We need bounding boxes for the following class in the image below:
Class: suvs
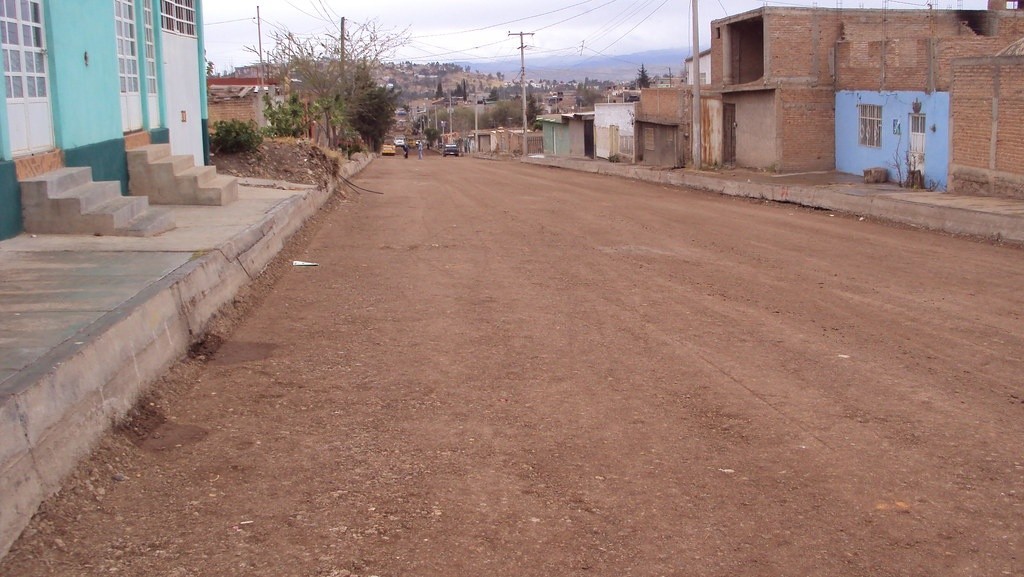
[442,144,460,157]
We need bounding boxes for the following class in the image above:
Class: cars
[397,107,408,115]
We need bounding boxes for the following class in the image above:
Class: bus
[395,134,406,147]
[381,135,396,156]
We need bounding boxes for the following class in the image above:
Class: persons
[417,140,423,158]
[403,142,409,158]
